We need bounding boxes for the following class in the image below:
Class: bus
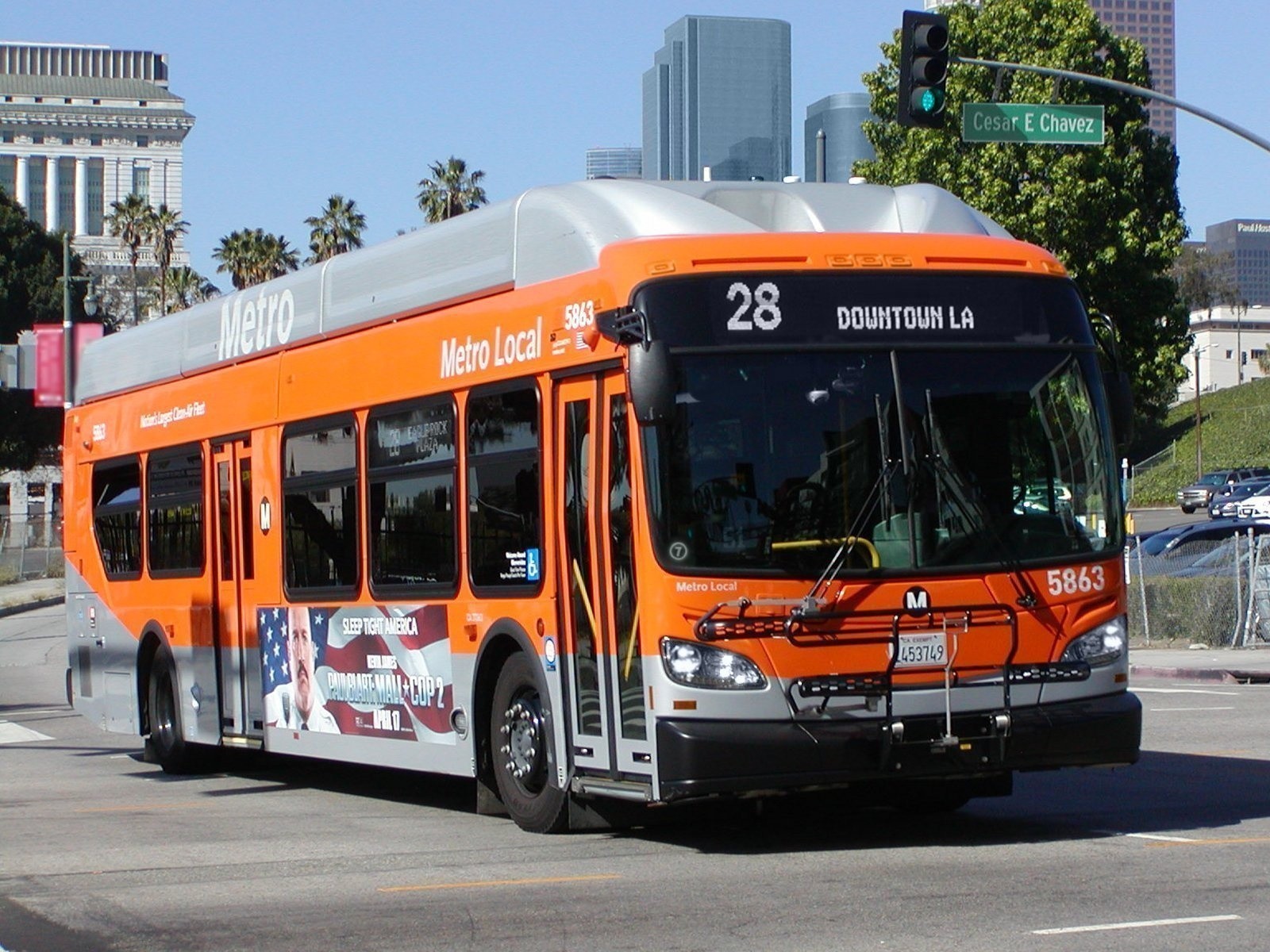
[64,177,1141,837]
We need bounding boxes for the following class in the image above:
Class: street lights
[1195,343,1219,481]
[1237,303,1262,386]
[54,231,99,417]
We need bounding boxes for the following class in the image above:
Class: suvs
[1128,515,1270,577]
[1177,465,1270,514]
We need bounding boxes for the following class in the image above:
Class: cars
[1236,485,1270,519]
[1208,480,1270,521]
[1212,475,1270,500]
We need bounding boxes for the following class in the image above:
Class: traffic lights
[899,7,951,130]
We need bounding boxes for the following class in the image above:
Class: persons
[889,439,979,516]
[262,607,342,734]
